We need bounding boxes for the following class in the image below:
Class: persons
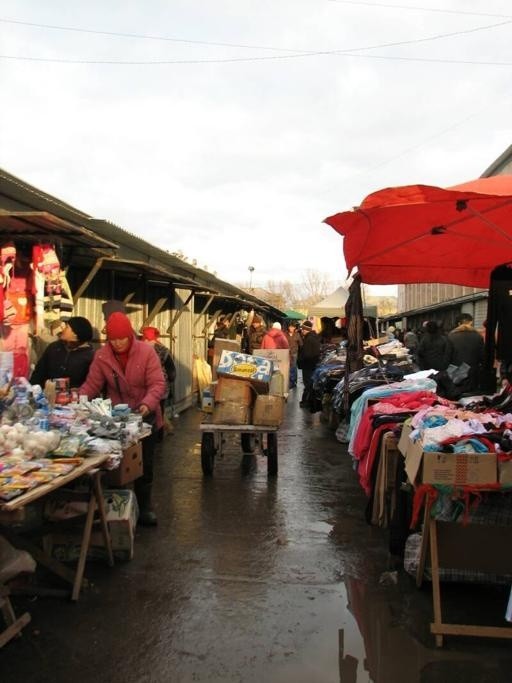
[385,319,455,372]
[439,311,490,395]
[74,310,167,526]
[136,325,178,455]
[206,313,336,409]
[476,319,499,394]
[27,316,95,386]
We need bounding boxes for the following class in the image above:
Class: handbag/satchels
[403,495,512,585]
[142,410,156,454]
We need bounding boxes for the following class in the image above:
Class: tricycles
[198,421,278,476]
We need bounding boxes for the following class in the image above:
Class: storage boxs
[211,348,290,425]
[395,423,511,485]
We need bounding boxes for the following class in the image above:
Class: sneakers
[138,502,158,526]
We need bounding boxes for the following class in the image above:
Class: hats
[253,316,261,322]
[142,327,155,342]
[0,241,16,287]
[272,321,282,330]
[301,321,312,331]
[106,312,132,339]
[68,317,92,341]
[0,299,17,327]
[456,313,472,323]
[32,244,61,273]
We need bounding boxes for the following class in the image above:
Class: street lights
[247,265,255,293]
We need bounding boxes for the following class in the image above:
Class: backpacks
[161,352,170,400]
